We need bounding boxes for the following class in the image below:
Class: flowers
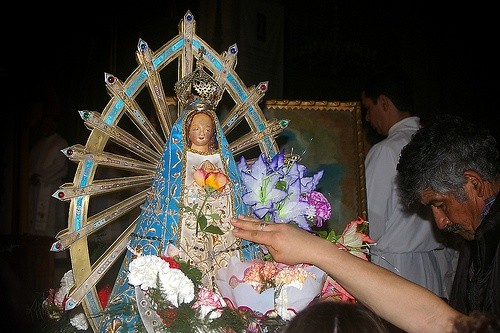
[39,146,399,333]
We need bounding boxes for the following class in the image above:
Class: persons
[99,55,306,333]
[16,111,72,289]
[232,80,500,332]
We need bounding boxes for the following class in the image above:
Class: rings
[260,222,268,231]
